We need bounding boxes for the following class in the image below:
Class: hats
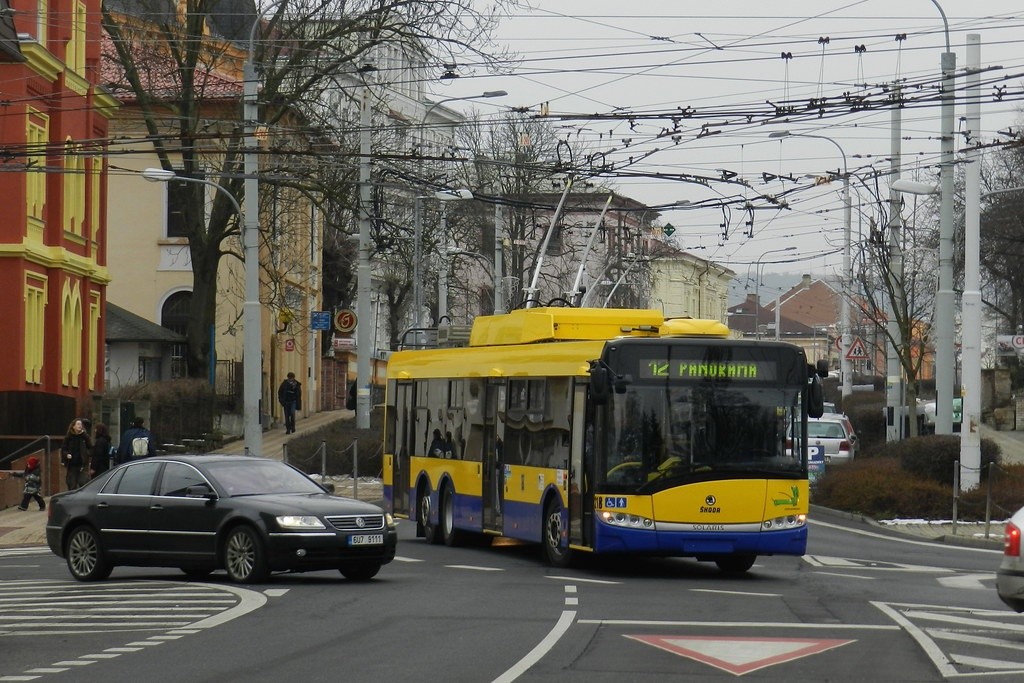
[27,455,40,469]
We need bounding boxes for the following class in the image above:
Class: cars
[820,368,966,436]
[46,457,397,585]
[788,413,860,468]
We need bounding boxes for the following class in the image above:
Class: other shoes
[18,507,27,511]
[286,429,290,434]
[40,505,46,510]
[292,428,296,433]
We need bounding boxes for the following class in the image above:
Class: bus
[382,176,817,576]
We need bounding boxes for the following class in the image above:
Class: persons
[91,423,111,478]
[496,435,504,468]
[119,417,156,464]
[444,432,456,458]
[278,372,302,434]
[427,429,446,458]
[586,399,641,462]
[9,456,46,511]
[63,418,92,490]
[350,378,357,415]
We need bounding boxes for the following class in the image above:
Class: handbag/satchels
[347,398,356,410]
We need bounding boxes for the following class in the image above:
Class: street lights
[635,200,691,310]
[268,309,297,429]
[405,89,508,352]
[769,288,810,343]
[143,165,262,461]
[756,246,798,339]
[768,130,853,408]
[892,177,953,441]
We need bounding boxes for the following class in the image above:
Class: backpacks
[105,439,118,460]
[132,429,149,455]
